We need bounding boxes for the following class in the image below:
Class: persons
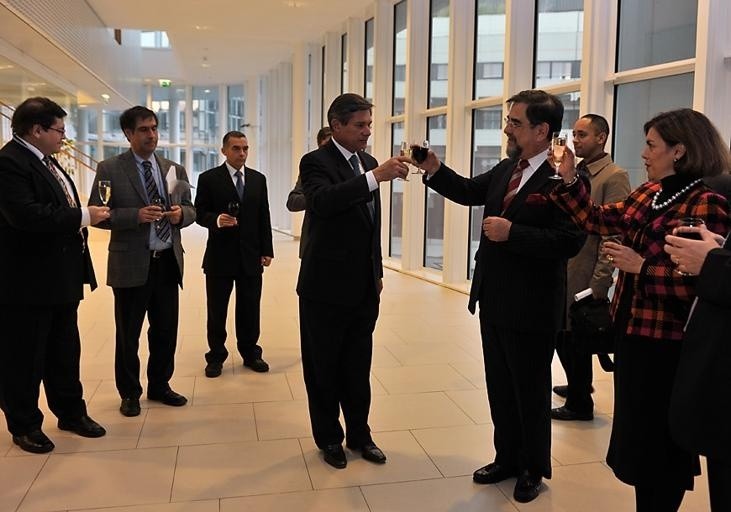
[551,114,632,421]
[296,93,415,468]
[286,127,334,260]
[410,90,590,503]
[664,224,731,512]
[194,131,274,377]
[87,106,196,416]
[0,97,110,454]
[547,108,731,512]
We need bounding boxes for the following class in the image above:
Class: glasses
[49,127,65,136]
[504,118,534,129]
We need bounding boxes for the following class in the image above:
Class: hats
[143,161,170,242]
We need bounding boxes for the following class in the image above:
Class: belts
[153,251,162,258]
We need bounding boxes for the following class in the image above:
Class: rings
[608,256,614,261]
[675,257,680,264]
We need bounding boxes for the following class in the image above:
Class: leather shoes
[58,414,105,437]
[550,386,593,421]
[119,395,140,416]
[355,441,386,462]
[514,467,542,503]
[243,357,269,372]
[13,430,54,453]
[147,390,187,406]
[473,461,518,484]
[323,444,346,468]
[205,362,222,377]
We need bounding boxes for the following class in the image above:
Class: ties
[350,155,374,222]
[44,157,86,253]
[500,160,530,216]
[234,171,244,202]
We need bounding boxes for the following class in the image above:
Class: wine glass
[674,218,706,278]
[396,142,414,182]
[548,132,568,180]
[152,199,165,230]
[410,141,429,175]
[99,180,112,208]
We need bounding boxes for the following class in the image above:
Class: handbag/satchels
[569,294,618,353]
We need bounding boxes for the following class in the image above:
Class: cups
[228,201,240,217]
